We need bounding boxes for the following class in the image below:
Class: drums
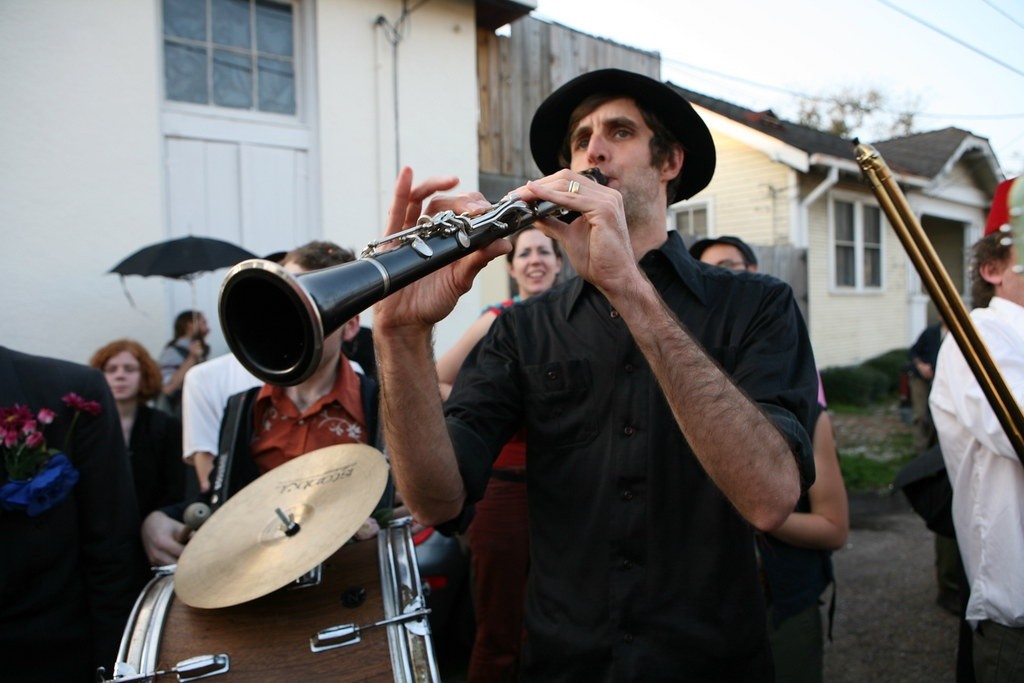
[107,520,445,683]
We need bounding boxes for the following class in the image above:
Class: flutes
[216,166,605,390]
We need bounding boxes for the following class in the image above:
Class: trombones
[849,142,1024,476]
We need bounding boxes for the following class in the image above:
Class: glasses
[717,260,751,269]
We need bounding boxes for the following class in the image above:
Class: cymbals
[169,440,393,612]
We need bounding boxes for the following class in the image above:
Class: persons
[170,249,430,548]
[381,65,828,683]
[152,308,214,417]
[912,172,1023,683]
[1,347,147,683]
[433,218,849,683]
[85,339,186,513]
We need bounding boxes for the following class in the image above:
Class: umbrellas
[104,234,263,316]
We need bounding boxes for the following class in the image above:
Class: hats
[530,69,715,207]
[690,236,757,265]
[984,175,1024,238]
